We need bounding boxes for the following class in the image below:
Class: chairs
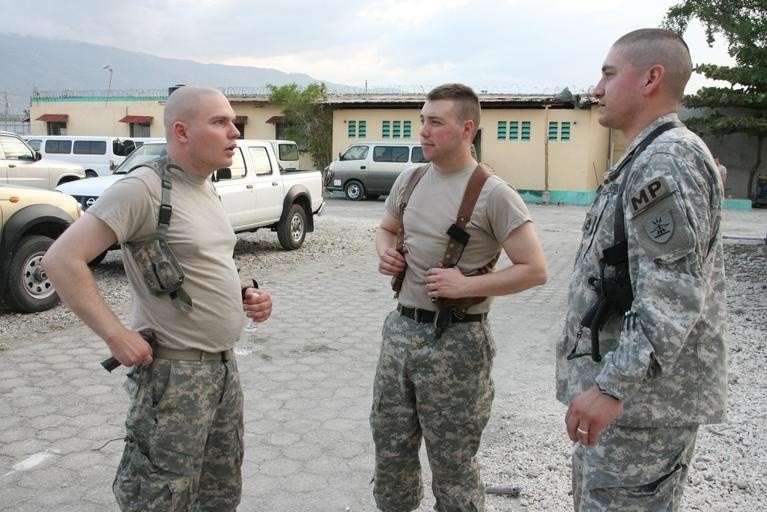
[374,150,391,161]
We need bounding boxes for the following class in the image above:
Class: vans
[322,140,477,202]
[26,137,136,178]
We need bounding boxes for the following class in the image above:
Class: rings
[576,427,589,434]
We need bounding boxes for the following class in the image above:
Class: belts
[151,348,233,362]
[395,302,488,323]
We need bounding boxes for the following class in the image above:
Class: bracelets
[241,278,259,300]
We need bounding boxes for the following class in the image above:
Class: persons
[555,28,725,512]
[369,84,548,512]
[712,152,728,191]
[38,86,272,512]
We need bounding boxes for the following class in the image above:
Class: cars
[0,129,87,195]
[0,181,108,316]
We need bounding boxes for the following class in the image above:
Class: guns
[580,277,635,331]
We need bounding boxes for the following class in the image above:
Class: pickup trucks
[53,139,324,251]
